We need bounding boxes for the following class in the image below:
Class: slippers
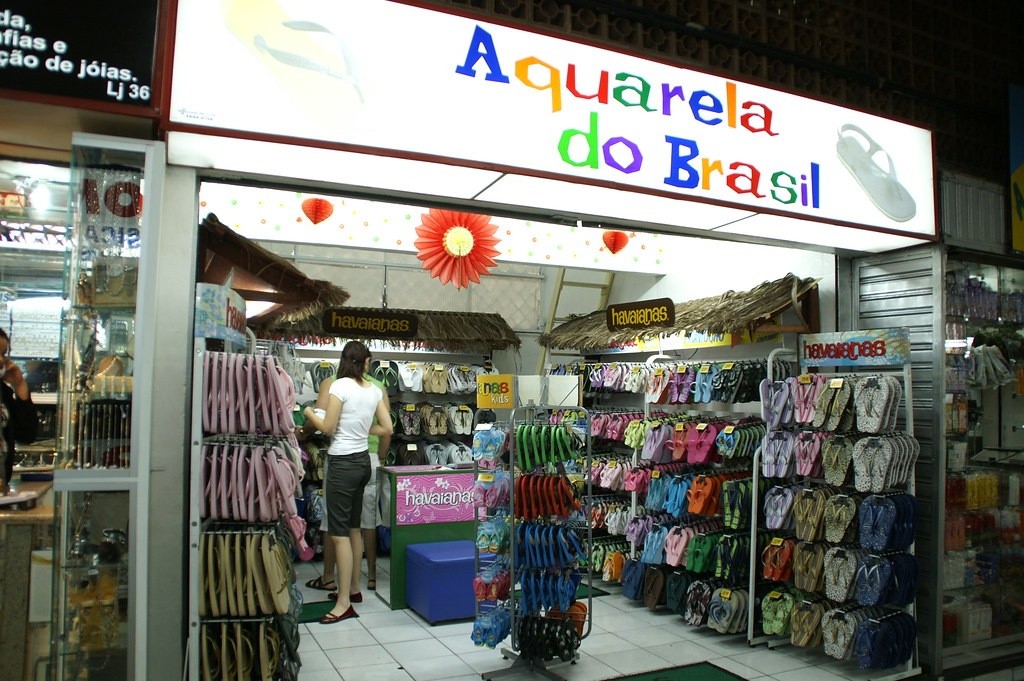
[471,426,587,662]
[551,362,765,633]
[196,352,313,680]
[759,373,921,672]
[281,341,499,480]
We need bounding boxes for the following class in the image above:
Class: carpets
[510,582,611,603]
[603,660,749,681]
[297,599,360,623]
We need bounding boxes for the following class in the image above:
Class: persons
[305,340,393,623]
[0,327,38,499]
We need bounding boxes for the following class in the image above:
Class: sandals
[305,575,337,591]
[367,579,376,590]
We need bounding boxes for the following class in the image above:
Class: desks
[0,482,73,681]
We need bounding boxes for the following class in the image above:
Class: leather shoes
[328,593,362,603]
[320,604,354,624]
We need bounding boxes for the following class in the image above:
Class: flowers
[415,207,504,289]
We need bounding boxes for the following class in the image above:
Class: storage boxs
[29,549,52,621]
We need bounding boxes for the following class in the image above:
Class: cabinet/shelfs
[246,305,520,560]
[850,247,1024,681]
[49,135,168,681]
[547,276,822,647]
[184,212,351,681]
[744,328,923,681]
[474,373,594,681]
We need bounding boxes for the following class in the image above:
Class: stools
[404,540,496,628]
